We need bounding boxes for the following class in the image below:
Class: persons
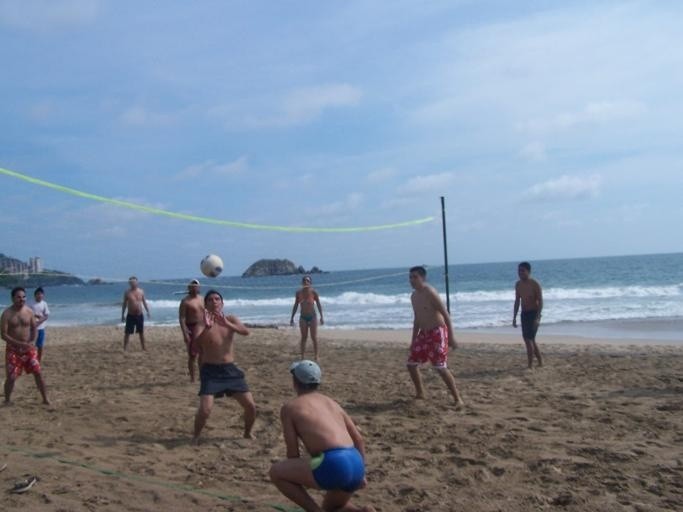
[264,358,369,511]
[176,278,205,382]
[28,288,49,364]
[510,258,545,371]
[0,286,55,407]
[118,276,151,353]
[284,273,326,362]
[189,289,258,443]
[402,263,464,410]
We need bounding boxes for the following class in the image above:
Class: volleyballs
[200,255,223,278]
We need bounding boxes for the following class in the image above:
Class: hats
[187,279,200,288]
[290,359,321,384]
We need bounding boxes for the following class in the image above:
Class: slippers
[11,476,37,493]
